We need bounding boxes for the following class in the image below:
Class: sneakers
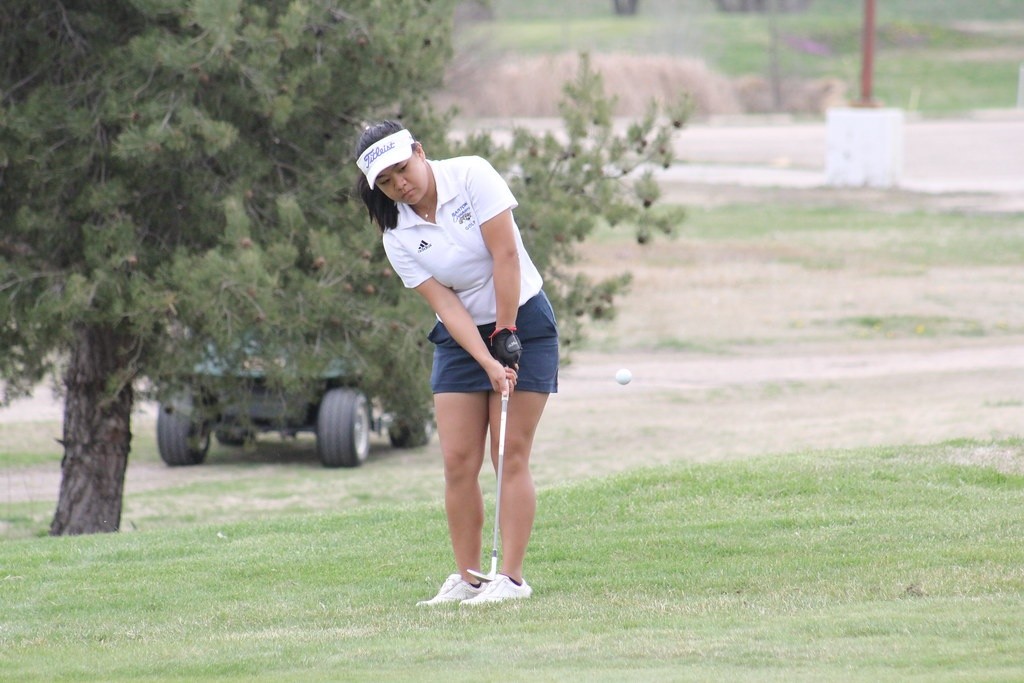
[415,573,489,608]
[459,573,533,609]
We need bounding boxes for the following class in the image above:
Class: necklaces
[401,164,436,220]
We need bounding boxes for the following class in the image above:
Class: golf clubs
[466,363,512,584]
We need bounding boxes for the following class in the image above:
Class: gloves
[489,327,523,374]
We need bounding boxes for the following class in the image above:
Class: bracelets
[495,325,518,331]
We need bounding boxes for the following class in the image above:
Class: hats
[356,128,415,191]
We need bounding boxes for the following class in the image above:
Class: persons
[355,120,560,609]
